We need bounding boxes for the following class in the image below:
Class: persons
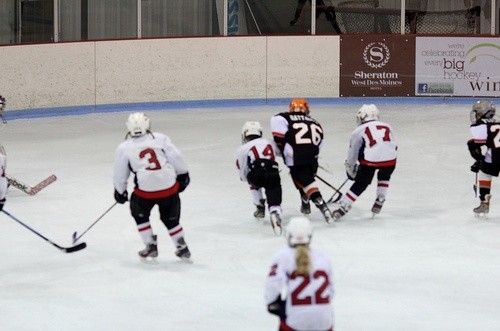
[0,96,9,211]
[266,217,336,331]
[236,121,284,236]
[270,99,335,225]
[467,98,500,213]
[110,112,191,259]
[331,104,398,221]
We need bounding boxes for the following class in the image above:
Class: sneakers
[315,196,335,225]
[253,199,266,224]
[300,203,311,214]
[175,236,192,262]
[473,194,491,220]
[331,205,347,223]
[371,196,385,219]
[138,234,158,261]
[270,209,283,238]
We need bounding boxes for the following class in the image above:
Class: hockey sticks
[315,174,342,203]
[2,209,87,254]
[73,201,117,244]
[328,178,349,202]
[6,173,58,197]
[473,172,478,197]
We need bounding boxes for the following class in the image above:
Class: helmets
[241,121,263,145]
[125,112,150,136]
[472,99,496,119]
[290,98,310,115]
[357,103,380,123]
[286,216,311,247]
[0,95,6,117]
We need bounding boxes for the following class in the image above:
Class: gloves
[346,172,355,181]
[268,293,286,319]
[176,172,190,193]
[115,188,129,204]
